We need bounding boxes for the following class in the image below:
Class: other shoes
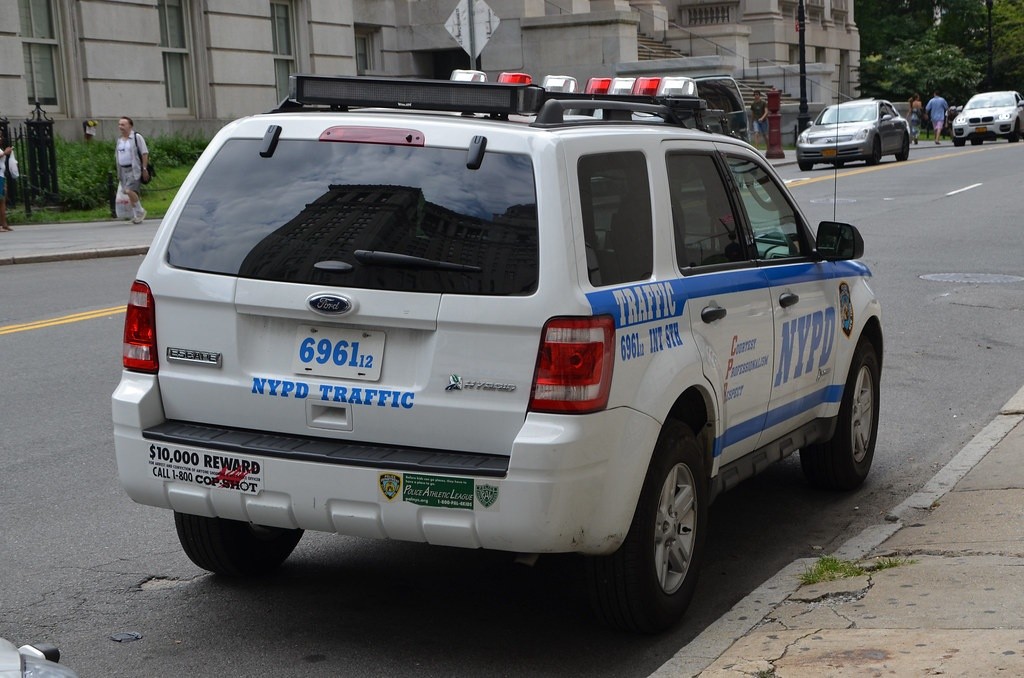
[0,226,13,232]
[135,208,147,222]
[128,218,142,224]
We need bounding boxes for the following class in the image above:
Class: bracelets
[143,168,147,171]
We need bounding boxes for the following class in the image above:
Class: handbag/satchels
[139,164,156,185]
[9,151,19,178]
[115,180,135,218]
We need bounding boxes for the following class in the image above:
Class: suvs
[109,59,887,638]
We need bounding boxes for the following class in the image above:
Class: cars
[795,96,911,172]
[951,91,1024,146]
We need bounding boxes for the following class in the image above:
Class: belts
[121,164,132,168]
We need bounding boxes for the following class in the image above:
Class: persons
[926,91,959,145]
[909,93,922,145]
[115,117,149,224]
[750,90,769,150]
[0,127,13,232]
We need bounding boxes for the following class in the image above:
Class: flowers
[84,120,98,126]
